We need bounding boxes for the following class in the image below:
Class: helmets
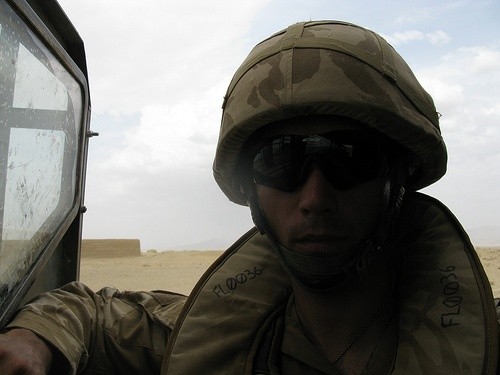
[210,20,446,204]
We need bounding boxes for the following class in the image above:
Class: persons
[0,21,500,375]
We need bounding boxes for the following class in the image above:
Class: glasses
[242,130,389,191]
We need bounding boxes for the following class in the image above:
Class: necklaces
[330,258,397,366]
